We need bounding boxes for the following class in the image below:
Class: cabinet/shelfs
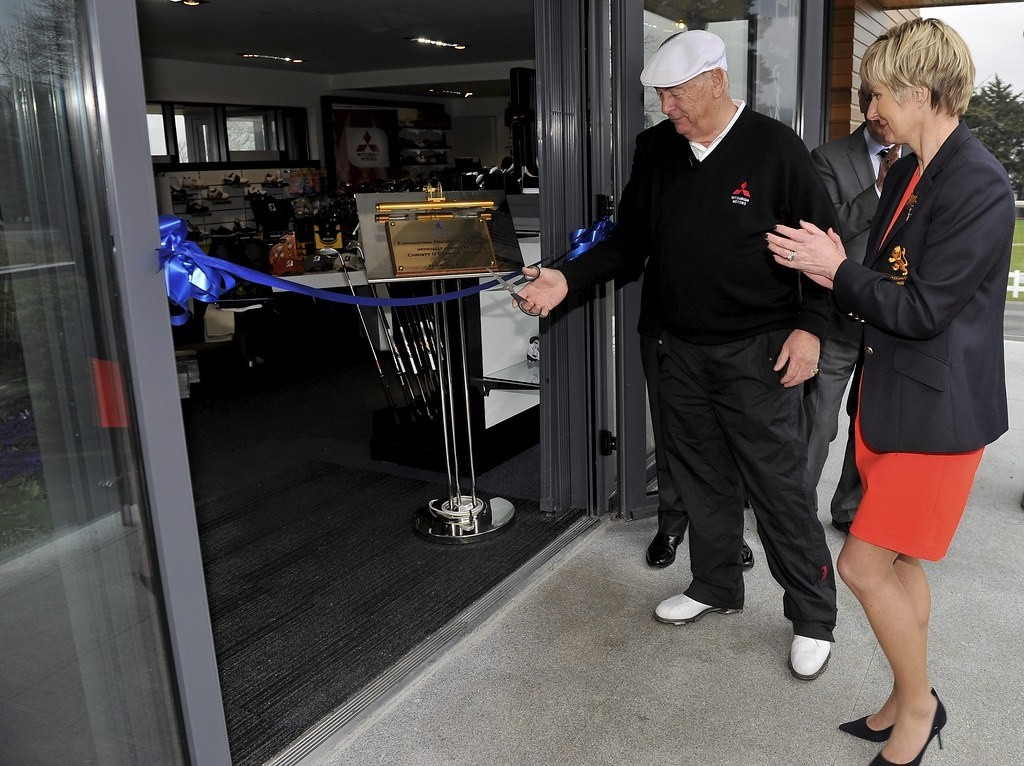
[403,118,453,179]
[152,158,330,260]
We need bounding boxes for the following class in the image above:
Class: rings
[786,250,796,262]
[810,368,819,374]
[887,161,890,169]
[885,156,890,163]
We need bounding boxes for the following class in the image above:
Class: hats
[639,30,727,87]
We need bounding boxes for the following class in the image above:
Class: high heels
[869,687,947,766]
[840,696,896,743]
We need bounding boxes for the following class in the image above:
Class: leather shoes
[654,594,742,625]
[832,519,852,535]
[788,634,831,681]
[741,539,754,570]
[646,530,685,568]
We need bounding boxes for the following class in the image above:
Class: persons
[801,53,924,539]
[512,29,853,682]
[635,242,755,571]
[760,16,1016,766]
